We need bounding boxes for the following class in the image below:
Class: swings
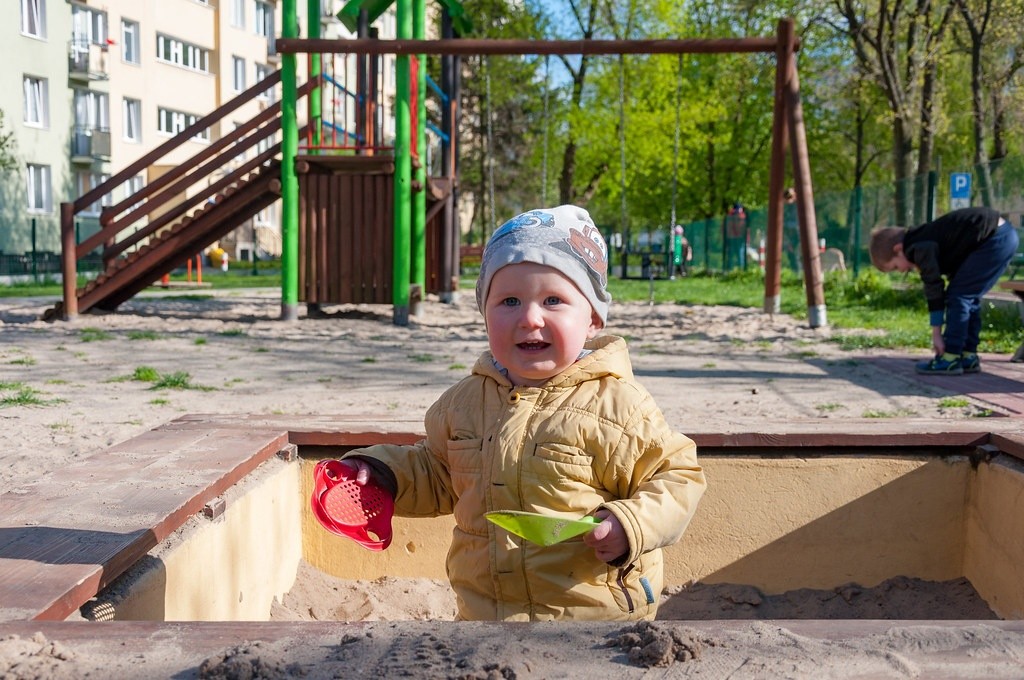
[479,54,552,234]
[619,53,683,281]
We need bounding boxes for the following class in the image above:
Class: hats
[473,203,612,327]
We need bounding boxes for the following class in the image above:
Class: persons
[326,204,708,622]
[869,206,1019,375]
[641,224,693,280]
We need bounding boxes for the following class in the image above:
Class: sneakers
[962,351,981,372]
[915,351,966,375]
